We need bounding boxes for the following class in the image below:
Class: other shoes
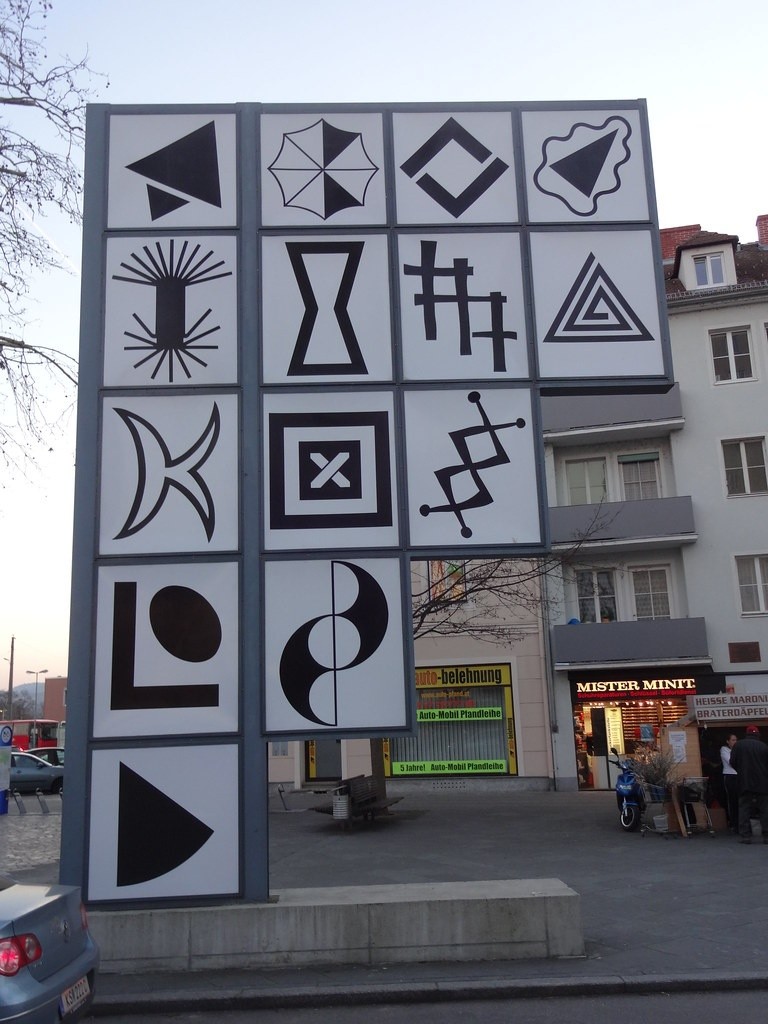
[763,835,768,844]
[735,834,753,844]
[727,827,737,836]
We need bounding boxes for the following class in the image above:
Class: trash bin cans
[333,795,351,819]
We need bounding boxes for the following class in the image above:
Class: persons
[730,724,768,844]
[720,732,751,836]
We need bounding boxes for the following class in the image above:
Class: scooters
[607,747,648,832]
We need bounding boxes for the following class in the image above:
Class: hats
[746,725,761,733]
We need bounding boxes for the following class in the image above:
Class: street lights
[26,670,49,718]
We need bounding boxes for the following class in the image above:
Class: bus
[0,719,59,753]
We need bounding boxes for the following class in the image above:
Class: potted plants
[600,607,609,623]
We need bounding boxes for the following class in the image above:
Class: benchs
[349,774,404,828]
[307,774,364,828]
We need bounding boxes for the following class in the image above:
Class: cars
[10,752,64,795]
[23,746,66,767]
[0,872,103,1024]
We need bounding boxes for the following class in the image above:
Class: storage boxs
[652,814,668,830]
